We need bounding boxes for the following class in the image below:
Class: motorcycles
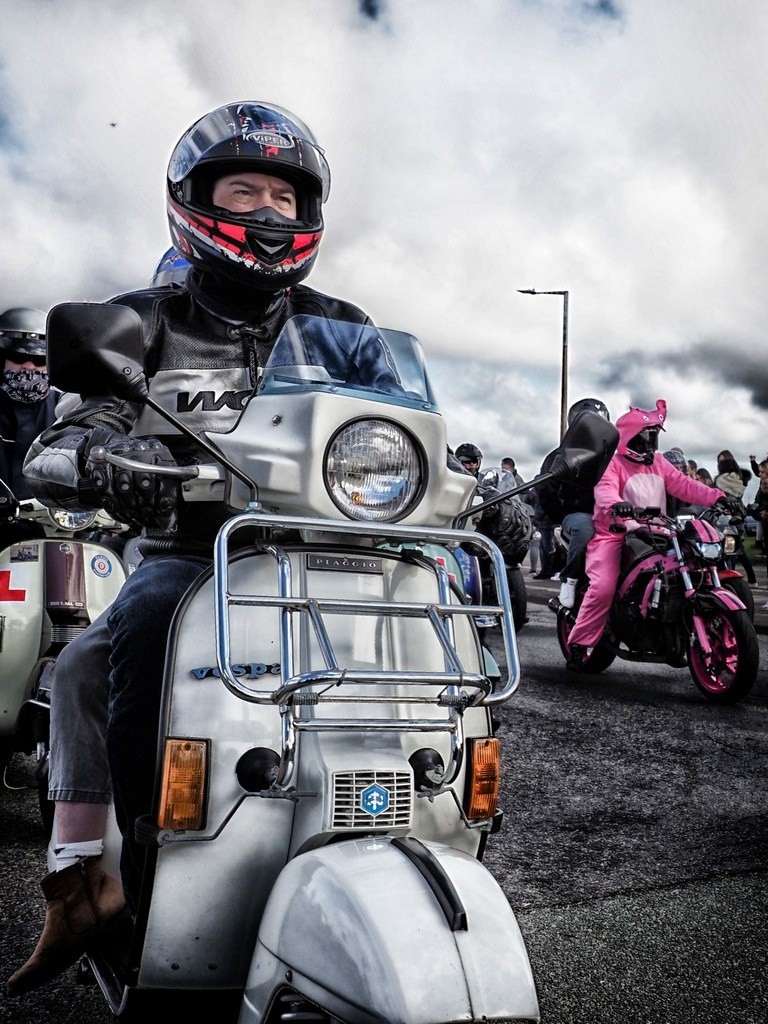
[0,292,767,1024]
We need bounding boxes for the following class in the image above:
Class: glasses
[0,349,49,368]
[463,458,477,464]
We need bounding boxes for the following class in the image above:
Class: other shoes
[528,569,537,576]
[748,582,758,588]
[533,573,551,580]
[564,645,584,682]
[8,851,125,997]
[550,572,561,581]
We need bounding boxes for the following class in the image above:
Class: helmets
[163,103,331,287]
[628,421,658,455]
[664,450,688,477]
[568,399,610,429]
[456,443,483,475]
[0,307,49,357]
[152,248,192,284]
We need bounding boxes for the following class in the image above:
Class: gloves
[79,424,181,528]
[717,496,740,517]
[458,485,535,569]
[612,502,633,518]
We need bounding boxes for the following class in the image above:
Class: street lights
[516,288,569,450]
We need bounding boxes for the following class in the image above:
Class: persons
[663,447,768,589]
[447,443,566,578]
[0,102,425,999]
[542,398,738,670]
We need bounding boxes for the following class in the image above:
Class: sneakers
[559,577,578,608]
